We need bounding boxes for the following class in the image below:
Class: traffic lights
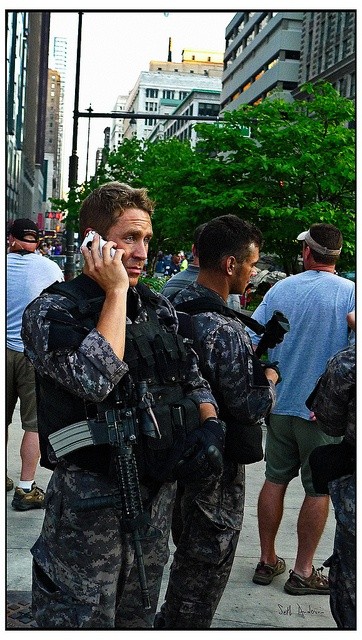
[44,211,62,221]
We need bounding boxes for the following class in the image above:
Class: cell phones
[80,230,122,269]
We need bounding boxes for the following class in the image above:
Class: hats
[296,228,344,257]
[7,217,39,243]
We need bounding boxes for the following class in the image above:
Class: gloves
[263,359,282,384]
[178,416,227,478]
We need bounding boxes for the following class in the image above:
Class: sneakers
[283,565,331,596]
[5,475,14,491]
[252,554,286,586]
[11,482,45,510]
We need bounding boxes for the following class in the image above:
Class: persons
[19,179,229,630]
[6,219,66,512]
[160,222,243,312]
[306,344,356,628]
[164,249,188,276]
[171,213,284,629]
[37,238,62,255]
[244,221,354,594]
